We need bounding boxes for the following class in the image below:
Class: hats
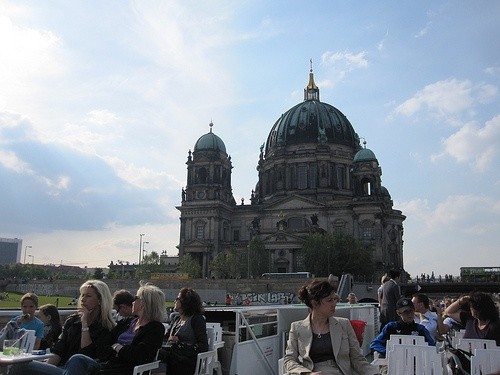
[395,299,414,308]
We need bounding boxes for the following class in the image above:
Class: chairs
[379,334,500,375]
[16,322,225,375]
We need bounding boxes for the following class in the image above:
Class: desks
[0,351,55,364]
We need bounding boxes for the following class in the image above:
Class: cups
[3,340,20,355]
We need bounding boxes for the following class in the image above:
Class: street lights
[24,245,34,264]
[139,233,149,264]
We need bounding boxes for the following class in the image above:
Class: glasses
[176,297,180,301]
[117,306,121,313]
[134,296,140,301]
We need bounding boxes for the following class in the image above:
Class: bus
[460,266,500,282]
[262,271,313,279]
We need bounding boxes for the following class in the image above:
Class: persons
[0,280,210,375]
[226,293,249,305]
[369,268,500,375]
[282,280,382,375]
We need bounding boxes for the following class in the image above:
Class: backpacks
[445,344,482,375]
